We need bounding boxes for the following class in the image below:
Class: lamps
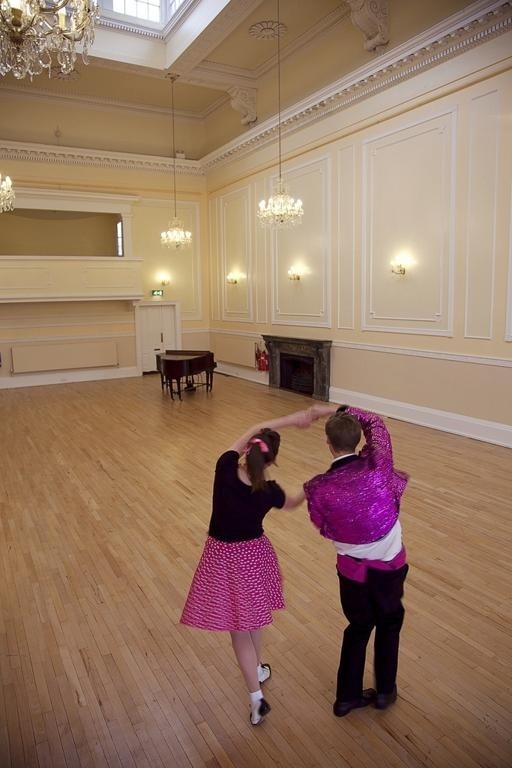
[1,176,16,213]
[257,1,305,230]
[0,1,100,83]
[391,254,407,277]
[157,270,170,286]
[288,261,306,281]
[158,73,195,249]
[228,269,245,285]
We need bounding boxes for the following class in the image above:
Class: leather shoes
[332,684,397,717]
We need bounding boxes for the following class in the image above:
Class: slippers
[259,663,271,685]
[249,699,271,726]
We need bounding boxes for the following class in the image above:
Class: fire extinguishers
[256,349,269,370]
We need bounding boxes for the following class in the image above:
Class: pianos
[156,350,217,401]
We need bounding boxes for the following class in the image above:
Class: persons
[180,410,313,728]
[302,404,411,717]
